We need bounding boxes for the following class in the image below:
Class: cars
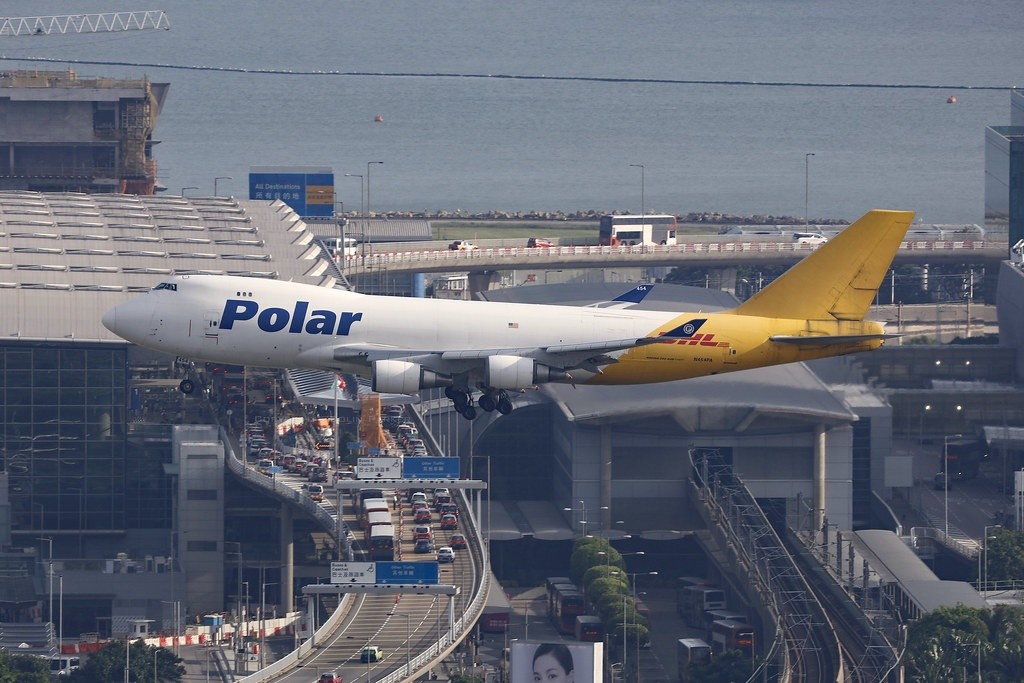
[527,237,556,248]
[194,362,469,683]
[449,240,478,252]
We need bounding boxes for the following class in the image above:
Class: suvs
[792,232,828,248]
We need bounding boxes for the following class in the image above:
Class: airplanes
[99,205,919,423]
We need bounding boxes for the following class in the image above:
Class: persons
[532,644,575,683]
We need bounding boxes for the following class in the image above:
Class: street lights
[318,190,338,263]
[979,536,996,595]
[368,160,383,244]
[629,164,645,254]
[344,174,365,268]
[562,501,659,683]
[945,433,962,545]
[805,153,816,233]
[983,524,1002,601]
[34,536,54,647]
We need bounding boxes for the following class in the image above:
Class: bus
[599,213,677,253]
[480,576,759,683]
[323,237,359,260]
